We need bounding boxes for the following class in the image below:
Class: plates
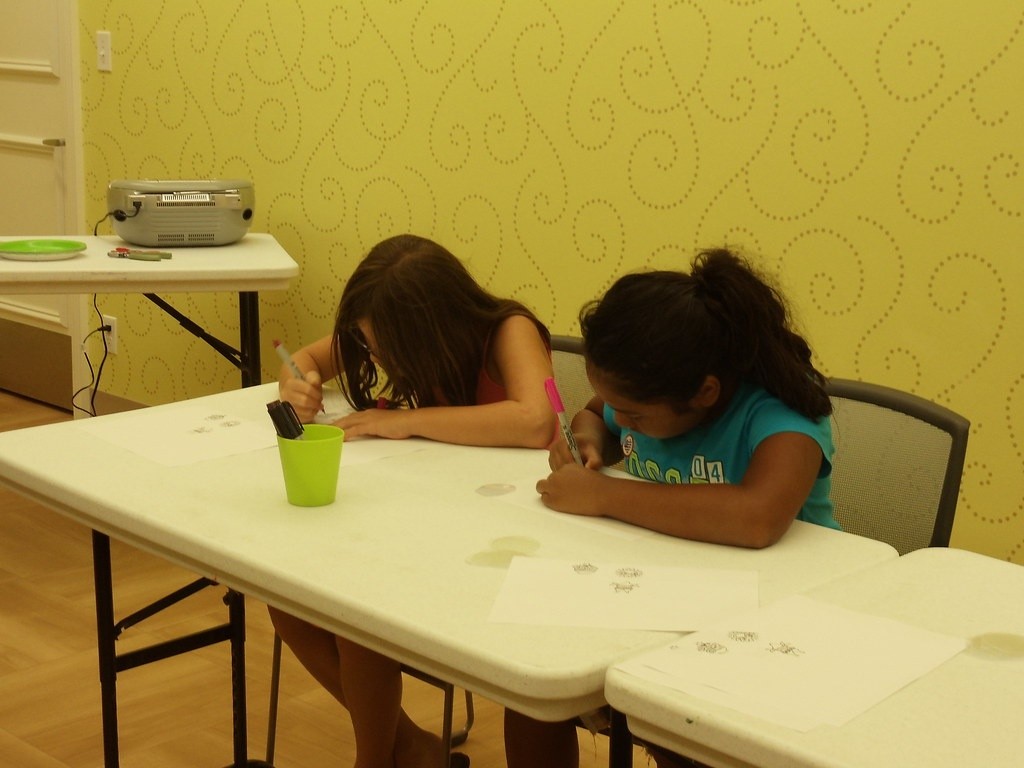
[0,239,87,261]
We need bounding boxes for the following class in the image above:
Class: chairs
[265,336,596,768]
[575,377,970,768]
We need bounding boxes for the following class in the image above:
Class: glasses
[346,327,383,362]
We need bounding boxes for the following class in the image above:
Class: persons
[267,236,556,767]
[503,249,843,768]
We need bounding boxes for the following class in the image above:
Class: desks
[0,234,300,389]
[606,545,1024,768]
[0,380,900,768]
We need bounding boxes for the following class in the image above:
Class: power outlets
[101,314,123,352]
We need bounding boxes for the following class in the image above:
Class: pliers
[108,247,173,260]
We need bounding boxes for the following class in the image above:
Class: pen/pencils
[266,397,306,442]
[272,338,326,416]
[544,376,585,468]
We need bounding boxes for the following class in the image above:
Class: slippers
[450,752,471,768]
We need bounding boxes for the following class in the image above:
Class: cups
[276,424,345,508]
